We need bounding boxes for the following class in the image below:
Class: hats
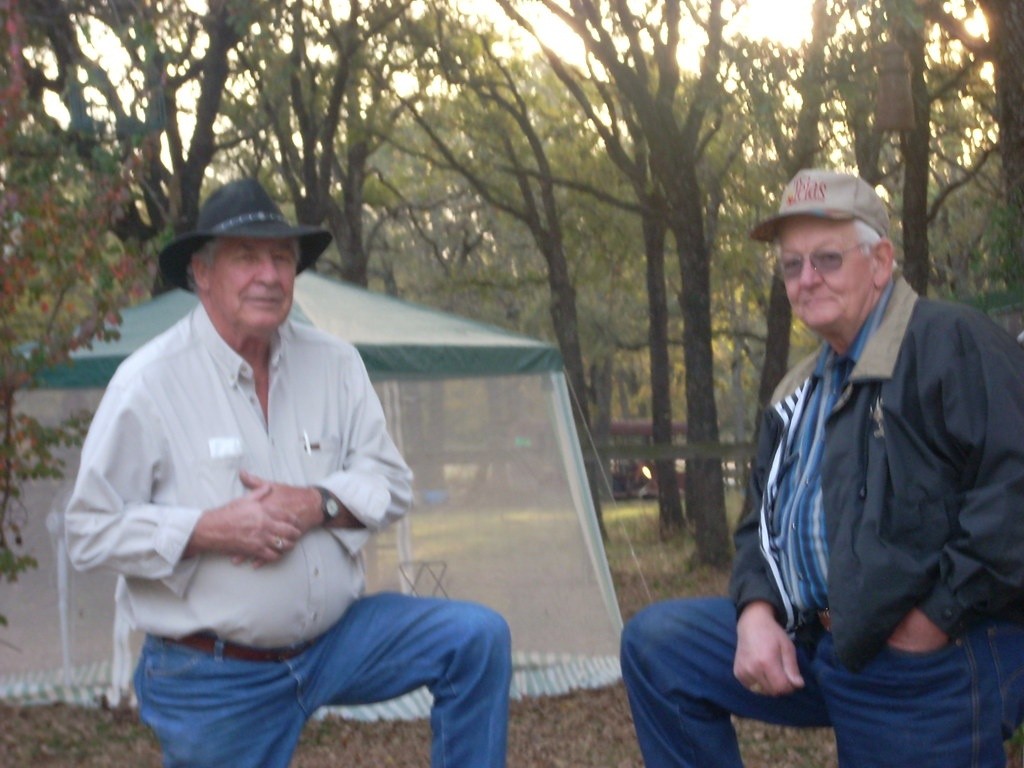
[752,169,890,244]
[159,178,333,294]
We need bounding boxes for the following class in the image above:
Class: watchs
[314,485,338,526]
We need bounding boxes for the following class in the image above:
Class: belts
[803,608,832,634]
[163,632,322,662]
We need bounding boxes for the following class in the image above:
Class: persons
[0,178,512,767]
[619,168,1024,768]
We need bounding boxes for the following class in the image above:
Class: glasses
[775,241,870,279]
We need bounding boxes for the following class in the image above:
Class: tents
[0,265,628,724]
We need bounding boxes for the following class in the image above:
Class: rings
[751,680,765,694]
[275,536,285,554]
